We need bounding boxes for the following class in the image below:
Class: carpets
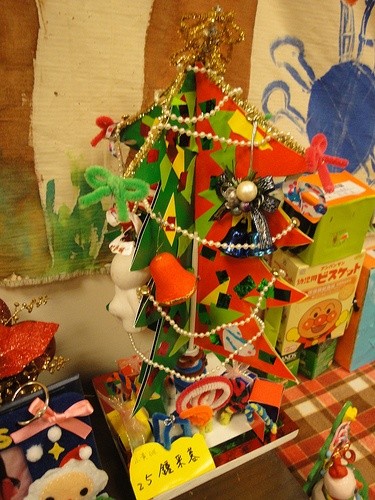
[273,362,375,500]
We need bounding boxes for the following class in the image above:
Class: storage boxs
[260,170,375,392]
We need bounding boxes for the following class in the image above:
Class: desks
[92,353,299,500]
